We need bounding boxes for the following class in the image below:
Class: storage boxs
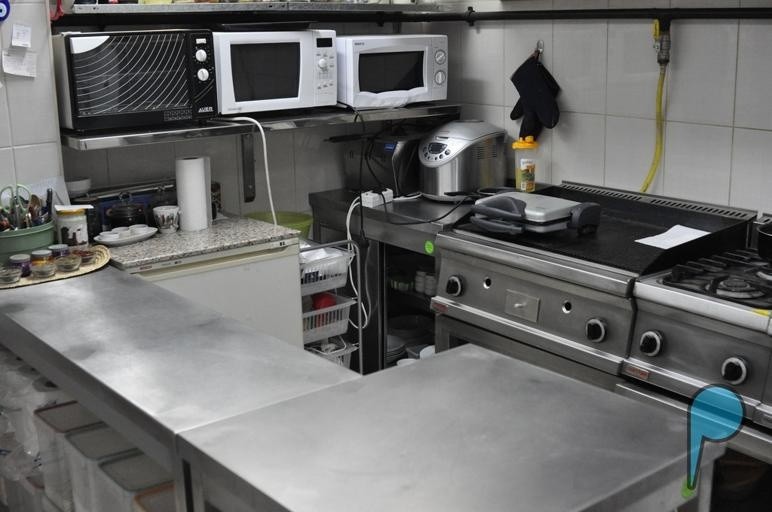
[28,399,177,508]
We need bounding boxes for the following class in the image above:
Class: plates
[93,225,160,246]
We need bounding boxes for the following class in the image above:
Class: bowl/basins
[243,210,314,242]
[129,223,149,235]
[0,265,22,284]
[71,250,96,264]
[27,261,57,279]
[99,231,120,241]
[54,255,82,271]
[383,263,443,369]
[63,176,92,199]
[112,227,130,237]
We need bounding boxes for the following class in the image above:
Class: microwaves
[337,35,450,113]
[50,30,218,134]
[212,31,338,116]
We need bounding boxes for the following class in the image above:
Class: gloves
[507,54,562,141]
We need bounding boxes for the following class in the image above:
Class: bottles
[30,249,52,264]
[47,244,69,258]
[512,135,539,194]
[55,208,88,253]
[8,253,31,277]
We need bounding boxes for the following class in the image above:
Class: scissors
[1,186,31,229]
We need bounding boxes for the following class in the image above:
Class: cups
[152,205,181,234]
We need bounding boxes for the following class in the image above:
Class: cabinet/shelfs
[297,237,372,375]
[54,0,464,155]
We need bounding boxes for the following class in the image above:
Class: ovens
[430,246,633,391]
[616,295,772,511]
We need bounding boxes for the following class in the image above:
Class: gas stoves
[659,242,772,313]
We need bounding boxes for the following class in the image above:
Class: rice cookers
[416,117,511,204]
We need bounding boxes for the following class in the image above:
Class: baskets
[300,290,357,347]
[297,234,357,299]
[303,337,360,370]
